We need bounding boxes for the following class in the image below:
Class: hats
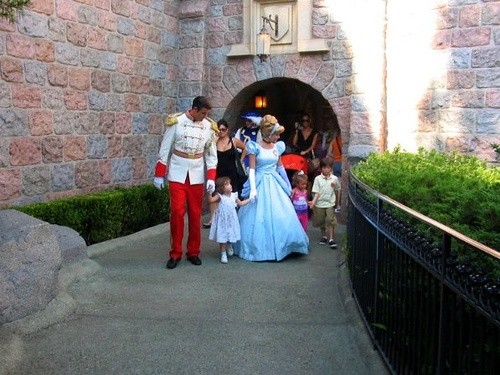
[240,112,262,126]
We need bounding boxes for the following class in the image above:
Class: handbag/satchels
[309,158,320,174]
[235,150,245,179]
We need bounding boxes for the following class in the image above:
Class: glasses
[219,127,227,132]
[301,119,309,123]
[198,110,209,117]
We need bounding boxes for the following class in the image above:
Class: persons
[241,114,293,260]
[289,170,312,233]
[154,96,218,269]
[231,112,342,213]
[310,159,341,247]
[202,118,247,227]
[207,177,251,263]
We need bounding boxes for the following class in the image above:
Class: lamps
[256,15,278,62]
[254,91,267,108]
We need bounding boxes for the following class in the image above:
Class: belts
[173,148,204,159]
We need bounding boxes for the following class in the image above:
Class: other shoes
[327,240,338,247]
[226,243,234,256]
[167,256,181,268]
[319,235,327,244]
[220,250,228,263]
[187,256,201,265]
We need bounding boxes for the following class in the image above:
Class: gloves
[248,167,258,203]
[206,179,215,194]
[278,165,294,197]
[153,176,166,190]
